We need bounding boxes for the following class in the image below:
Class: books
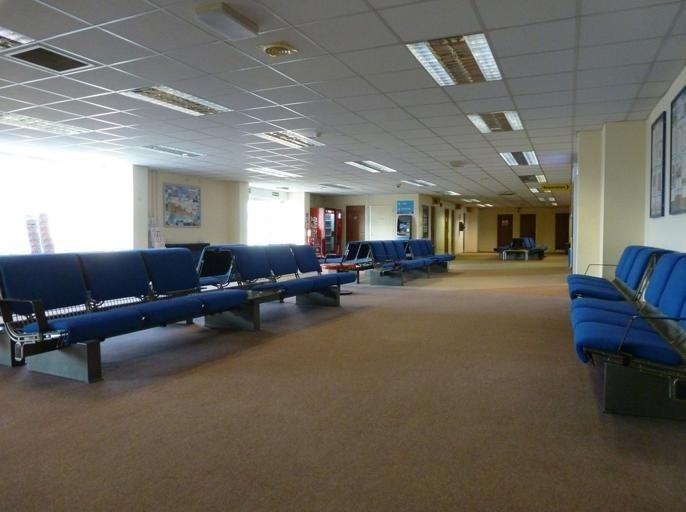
[150,228,165,248]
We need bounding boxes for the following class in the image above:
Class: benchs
[316,240,456,286]
[164,239,358,331]
[1,248,247,381]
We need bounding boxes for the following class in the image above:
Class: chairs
[493,235,548,261]
[566,245,686,421]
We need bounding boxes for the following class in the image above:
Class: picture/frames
[668,85,685,215]
[648,110,666,219]
